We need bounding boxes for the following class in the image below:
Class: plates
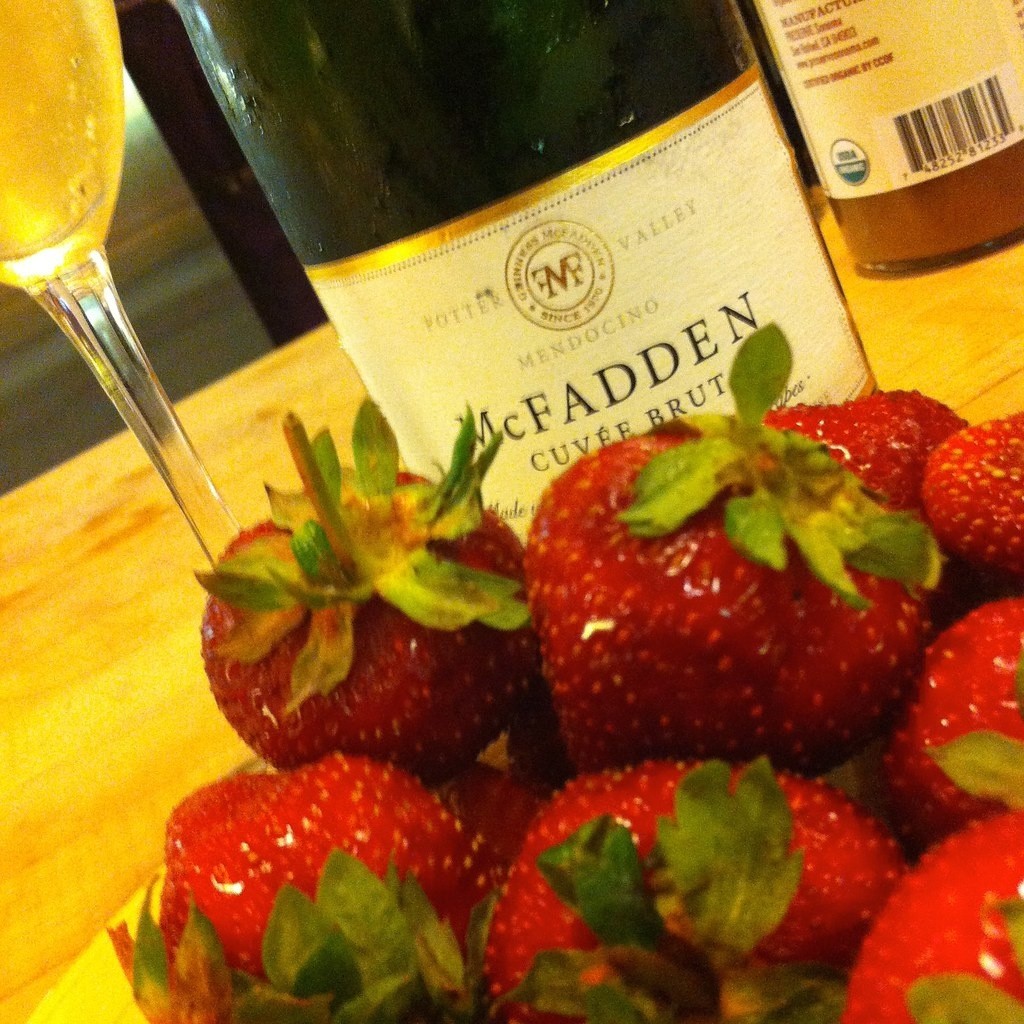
[26,866,171,1024]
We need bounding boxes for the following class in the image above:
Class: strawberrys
[144,390,1024,1024]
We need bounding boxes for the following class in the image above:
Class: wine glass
[0,0,237,570]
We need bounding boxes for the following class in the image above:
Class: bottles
[746,0,1024,282]
[175,0,877,528]
